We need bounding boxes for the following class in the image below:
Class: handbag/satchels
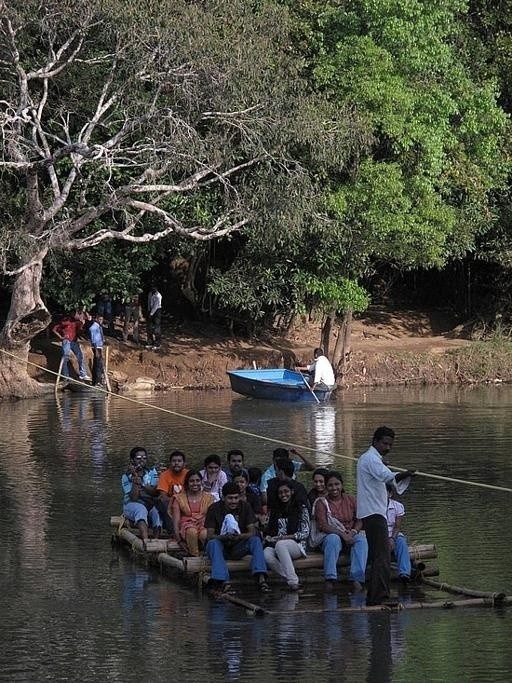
[308,517,346,547]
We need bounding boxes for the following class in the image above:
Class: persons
[52,281,163,388]
[121,445,413,598]
[294,347,336,392]
[355,425,419,605]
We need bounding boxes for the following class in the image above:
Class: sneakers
[290,585,304,593]
[400,575,411,587]
[349,581,363,590]
[325,580,337,590]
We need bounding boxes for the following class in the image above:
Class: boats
[225,368,338,401]
[109,513,511,618]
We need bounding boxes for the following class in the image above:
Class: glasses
[132,455,148,459]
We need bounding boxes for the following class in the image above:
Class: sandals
[222,583,237,595]
[260,582,273,592]
[365,596,400,606]
